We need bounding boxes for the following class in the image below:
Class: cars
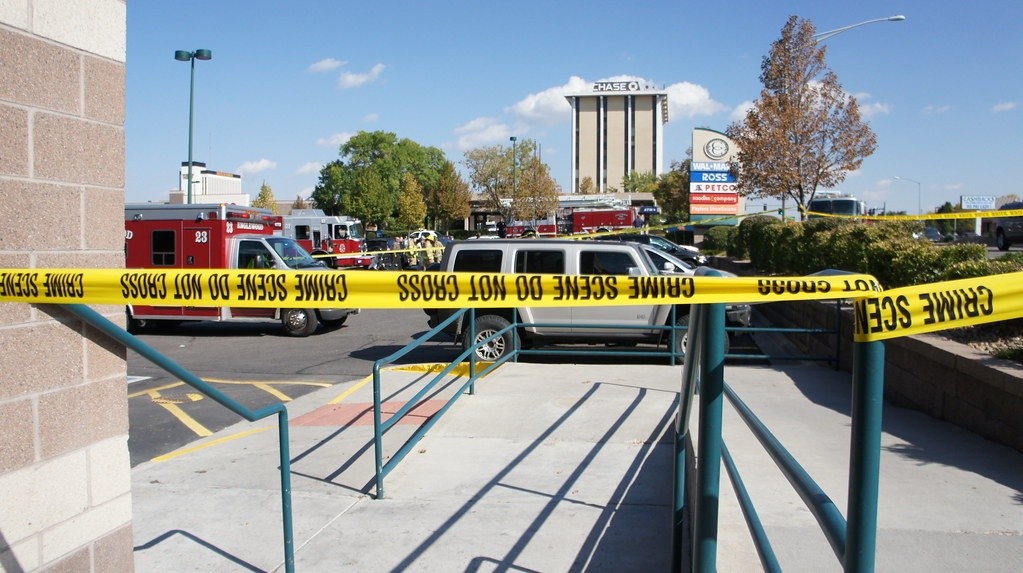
[923,227,944,244]
[368,239,393,263]
[987,202,1023,251]
[399,229,453,247]
[587,233,710,273]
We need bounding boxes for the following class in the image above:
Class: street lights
[174,48,212,204]
[893,176,921,216]
[781,14,906,221]
[509,135,517,198]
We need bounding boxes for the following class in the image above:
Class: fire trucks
[496,206,636,238]
[123,203,373,338]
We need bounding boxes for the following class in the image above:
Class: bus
[808,196,871,220]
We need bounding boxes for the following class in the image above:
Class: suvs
[424,233,755,367]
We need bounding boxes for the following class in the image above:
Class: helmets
[427,235,434,241]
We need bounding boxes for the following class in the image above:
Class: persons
[633,215,644,235]
[386,234,444,273]
